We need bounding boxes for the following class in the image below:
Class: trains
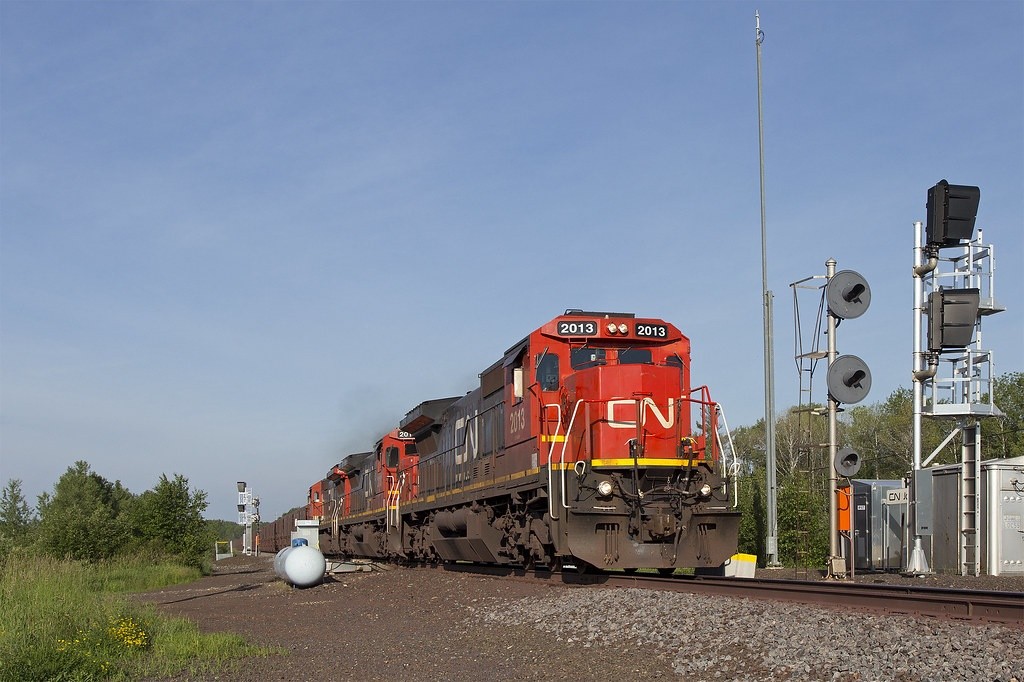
[233,308,744,576]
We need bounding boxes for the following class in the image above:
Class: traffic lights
[923,180,980,247]
[834,447,861,477]
[828,268,873,320]
[827,356,873,402]
[925,285,1009,352]
